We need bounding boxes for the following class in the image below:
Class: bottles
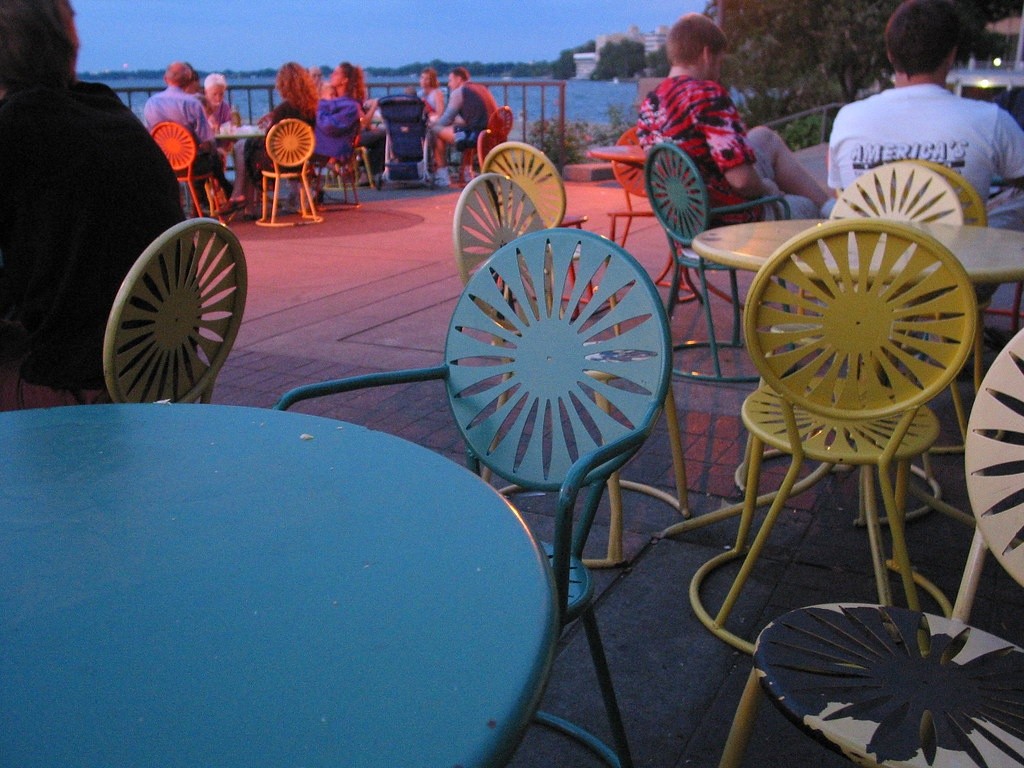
[229,95,240,129]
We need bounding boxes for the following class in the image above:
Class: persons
[0,0,202,411]
[636,12,838,248]
[142,62,380,221]
[404,67,498,187]
[827,0,1024,381]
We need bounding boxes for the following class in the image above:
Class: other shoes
[234,214,259,222]
[432,167,450,187]
[357,180,375,186]
[215,196,248,216]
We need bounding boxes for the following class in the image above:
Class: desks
[1,402,563,768]
[208,124,271,216]
[364,112,464,182]
[946,63,1024,102]
[689,207,1022,525]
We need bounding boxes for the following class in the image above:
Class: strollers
[377,95,434,189]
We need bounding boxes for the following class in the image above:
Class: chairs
[1,111,1024,767]
